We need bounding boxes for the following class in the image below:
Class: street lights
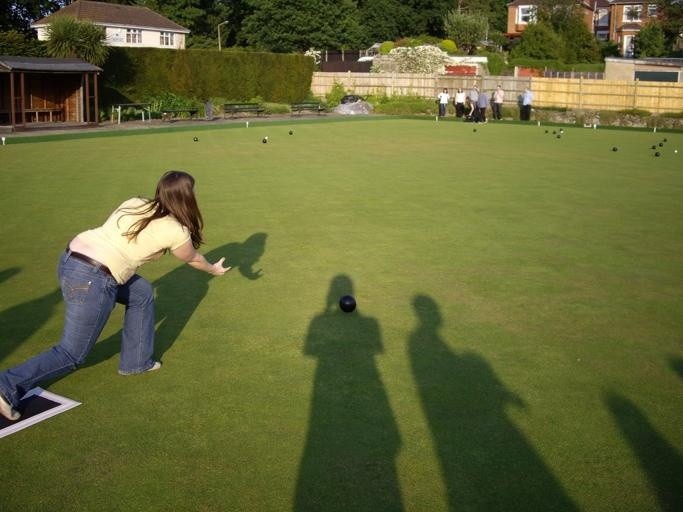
[217,20,229,52]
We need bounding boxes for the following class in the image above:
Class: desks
[109,102,152,125]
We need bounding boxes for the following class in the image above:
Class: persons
[436,87,450,117]
[453,85,489,125]
[491,85,505,121]
[519,87,534,121]
[0,170,232,421]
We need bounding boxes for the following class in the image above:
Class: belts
[66,243,112,276]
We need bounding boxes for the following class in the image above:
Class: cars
[342,95,365,105]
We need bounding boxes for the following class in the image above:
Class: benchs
[292,102,325,115]
[159,101,198,120]
[224,103,264,118]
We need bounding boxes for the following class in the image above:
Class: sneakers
[0,391,21,421]
[118,361,161,376]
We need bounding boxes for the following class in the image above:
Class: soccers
[674,150,678,153]
[655,152,660,157]
[652,145,656,149]
[193,137,198,141]
[339,295,356,313]
[473,129,477,133]
[545,130,548,133]
[664,139,667,142]
[289,131,293,135]
[613,147,617,151]
[659,143,663,147]
[262,136,268,143]
[553,129,564,138]
